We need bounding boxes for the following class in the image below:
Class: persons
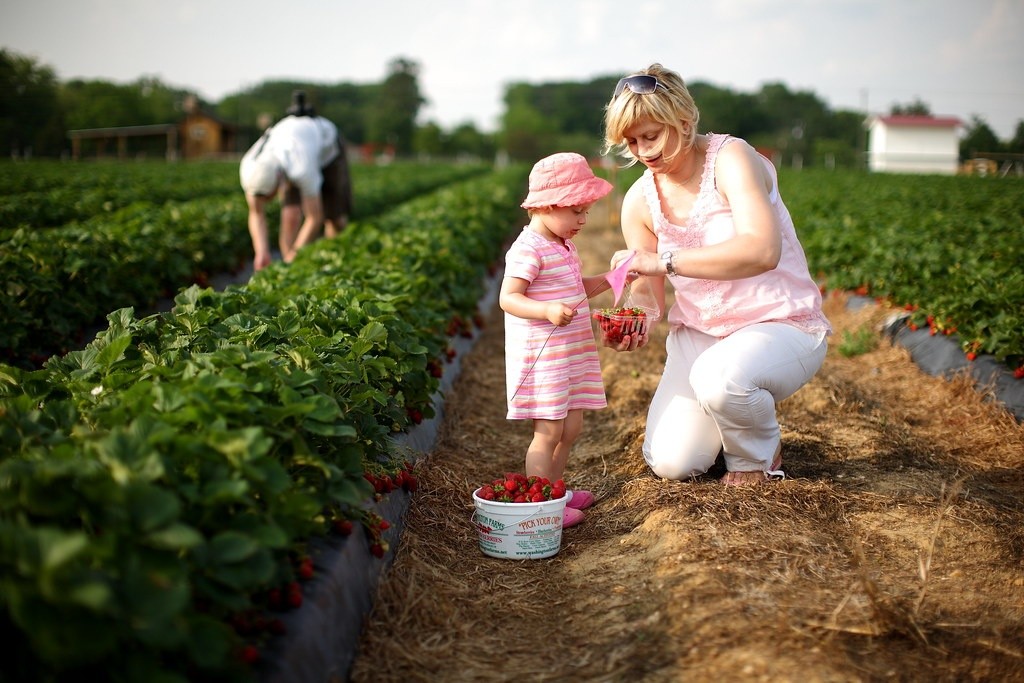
[498,152,639,525]
[597,63,836,491]
[236,113,353,274]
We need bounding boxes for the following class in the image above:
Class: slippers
[718,457,785,491]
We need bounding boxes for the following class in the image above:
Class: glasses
[609,75,682,100]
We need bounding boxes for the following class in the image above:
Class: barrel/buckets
[469,487,569,560]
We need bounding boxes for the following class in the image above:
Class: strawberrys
[234,458,420,636]
[405,312,487,424]
[816,273,1024,379]
[592,307,647,341]
[475,473,567,505]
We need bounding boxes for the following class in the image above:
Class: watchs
[661,250,677,279]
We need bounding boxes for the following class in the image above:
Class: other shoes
[560,507,585,529]
[564,487,593,509]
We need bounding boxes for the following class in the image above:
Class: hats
[518,151,616,219]
[240,155,284,198]
